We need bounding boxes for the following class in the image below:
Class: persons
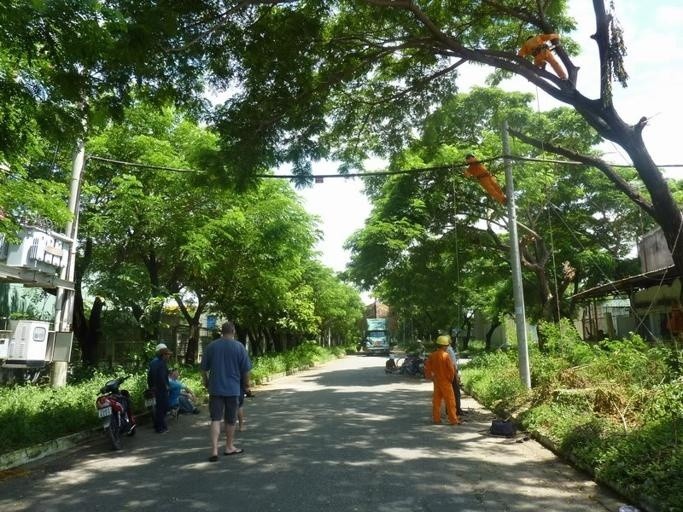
[424,335,464,426]
[383,355,396,374]
[166,367,199,414]
[443,335,467,415]
[234,378,247,433]
[147,342,171,436]
[197,320,250,461]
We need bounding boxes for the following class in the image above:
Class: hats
[156,343,173,357]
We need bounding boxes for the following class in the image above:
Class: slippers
[225,449,244,455]
[208,454,219,462]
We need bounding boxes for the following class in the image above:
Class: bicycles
[385,345,425,377]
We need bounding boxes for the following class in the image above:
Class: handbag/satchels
[490,420,516,438]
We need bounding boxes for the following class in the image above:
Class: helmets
[436,334,452,345]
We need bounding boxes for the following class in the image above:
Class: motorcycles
[96,375,136,450]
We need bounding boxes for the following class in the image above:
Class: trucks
[362,318,391,355]
